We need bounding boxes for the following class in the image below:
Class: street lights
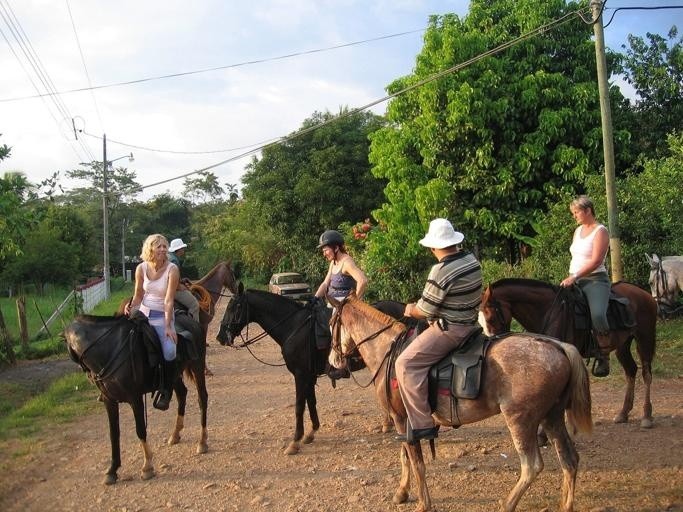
[104,135,135,300]
[122,215,134,281]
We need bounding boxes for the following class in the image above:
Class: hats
[419,218,464,249]
[168,238,189,255]
[316,229,345,248]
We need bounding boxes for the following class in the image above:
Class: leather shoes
[394,425,439,445]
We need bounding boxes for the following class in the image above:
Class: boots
[594,347,611,378]
[156,380,175,409]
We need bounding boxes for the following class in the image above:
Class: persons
[559,195,613,377]
[313,231,368,378]
[166,238,200,321]
[124,234,178,410]
[394,217,483,443]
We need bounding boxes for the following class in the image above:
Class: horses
[117,259,238,381]
[644,251,683,321]
[324,286,592,512]
[476,278,657,447]
[57,314,208,485]
[215,281,429,455]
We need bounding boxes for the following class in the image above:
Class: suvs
[269,272,313,302]
